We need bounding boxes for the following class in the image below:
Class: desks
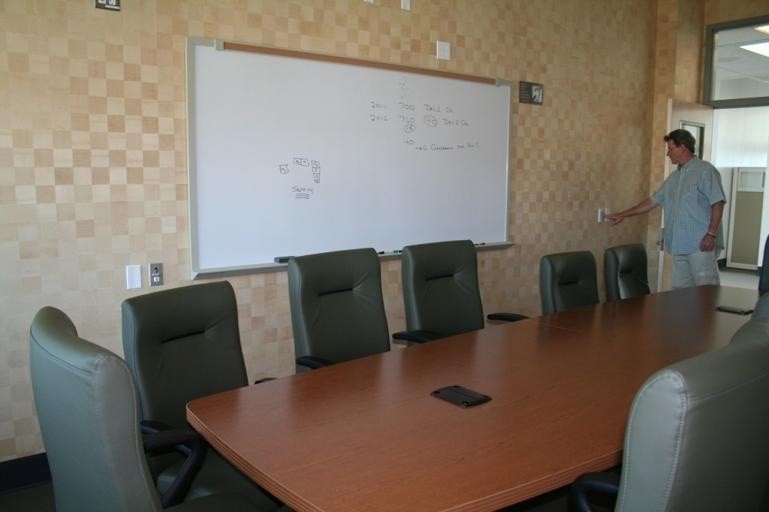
[186,285,760,512]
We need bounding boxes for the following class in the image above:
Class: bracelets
[707,231,716,238]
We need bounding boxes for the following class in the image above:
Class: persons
[603,128,727,290]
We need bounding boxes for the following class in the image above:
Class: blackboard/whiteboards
[186,36,514,282]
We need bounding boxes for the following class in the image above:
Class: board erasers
[274,257,294,263]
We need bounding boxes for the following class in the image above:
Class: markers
[394,250,401,252]
[376,252,385,254]
[474,243,486,245]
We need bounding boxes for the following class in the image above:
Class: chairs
[401,240,530,346]
[287,248,442,374]
[567,292,765,512]
[539,250,599,313]
[30,281,280,512]
[603,244,651,301]
